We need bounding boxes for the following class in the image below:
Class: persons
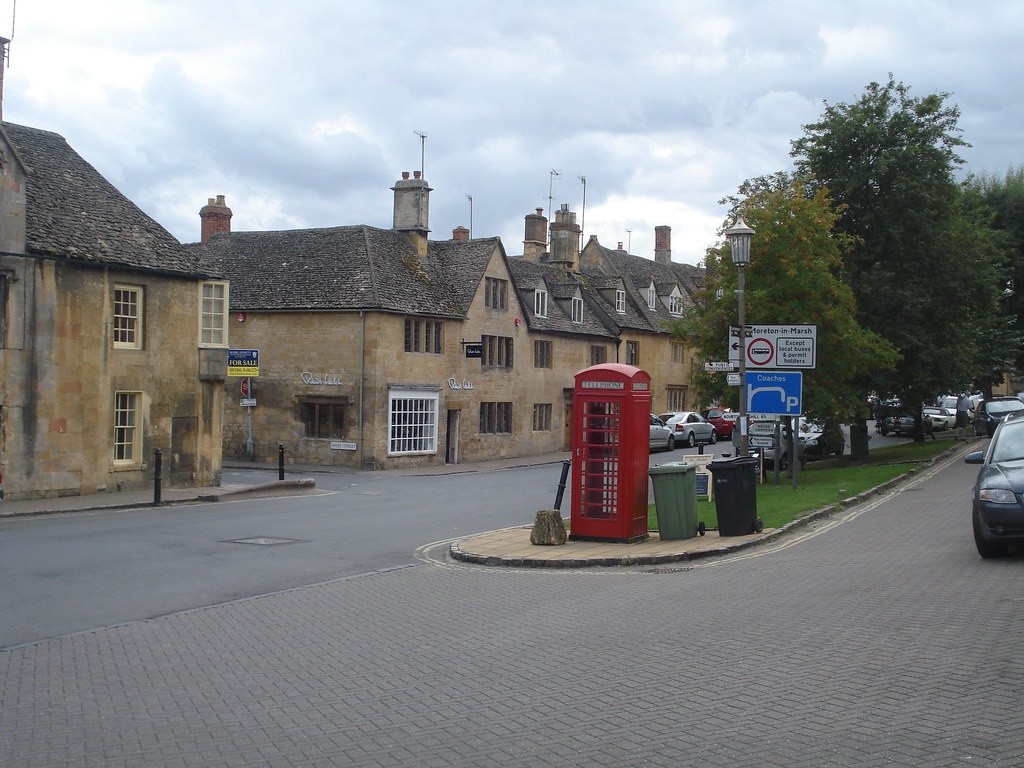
[954,389,976,440]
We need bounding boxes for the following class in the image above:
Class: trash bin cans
[647,461,705,541]
[706,455,763,537]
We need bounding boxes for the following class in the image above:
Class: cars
[791,417,845,459]
[737,424,808,470]
[971,398,1024,437]
[963,413,1024,559]
[878,413,931,437]
[921,407,957,431]
[701,408,736,440]
[606,413,676,454]
[659,412,719,447]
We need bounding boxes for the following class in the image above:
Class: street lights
[728,216,757,456]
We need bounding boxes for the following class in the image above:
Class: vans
[943,394,983,424]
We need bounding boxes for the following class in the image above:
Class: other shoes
[962,439,966,441]
[955,436,958,440]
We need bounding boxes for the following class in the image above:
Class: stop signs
[241,378,250,398]
